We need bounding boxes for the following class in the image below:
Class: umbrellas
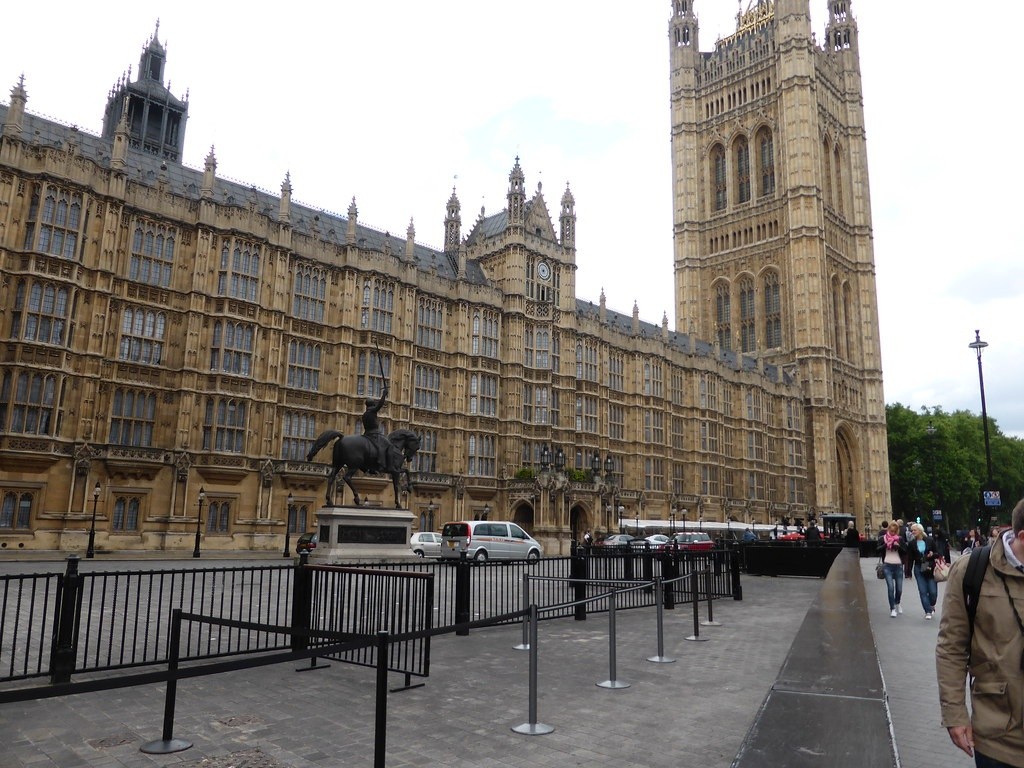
[780,532,824,541]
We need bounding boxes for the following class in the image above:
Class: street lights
[635,512,639,536]
[193,486,205,557]
[283,491,293,557]
[926,421,950,563]
[969,330,993,492]
[86,479,101,558]
[668,505,687,536]
[751,517,756,533]
[775,518,778,540]
[606,502,611,533]
[911,460,923,512]
[698,514,703,532]
[618,502,624,534]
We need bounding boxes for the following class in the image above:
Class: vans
[441,521,543,566]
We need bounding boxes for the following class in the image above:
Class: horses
[305,428,422,510]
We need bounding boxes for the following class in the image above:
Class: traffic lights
[976,506,984,526]
[916,513,921,524]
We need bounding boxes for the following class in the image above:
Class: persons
[805,522,822,546]
[743,528,758,541]
[876,521,907,617]
[960,529,999,555]
[936,498,1024,768]
[878,518,948,581]
[583,530,590,542]
[908,523,939,620]
[362,389,402,473]
[843,521,854,536]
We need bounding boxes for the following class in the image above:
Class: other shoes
[895,604,902,613]
[891,609,898,616]
[925,605,937,619]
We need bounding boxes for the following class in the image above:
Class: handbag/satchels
[919,552,950,582]
[876,564,884,579]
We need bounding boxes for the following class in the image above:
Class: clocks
[537,262,551,280]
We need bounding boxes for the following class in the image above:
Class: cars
[594,532,716,557]
[779,529,825,542]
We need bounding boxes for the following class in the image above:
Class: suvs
[296,533,317,553]
[408,531,442,558]
